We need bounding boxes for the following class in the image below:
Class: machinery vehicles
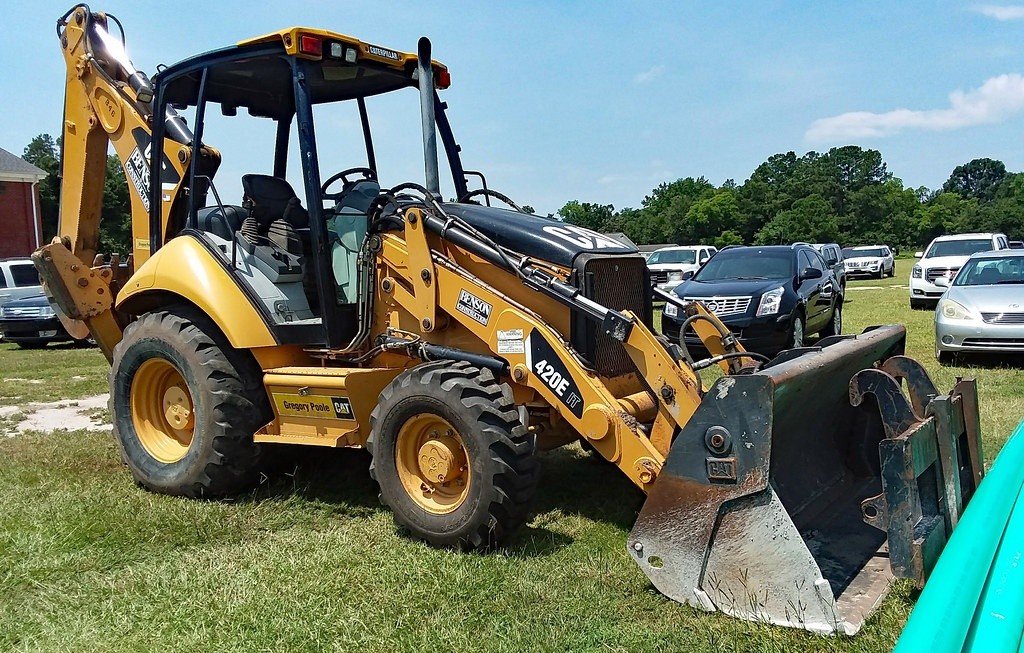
[31,6,985,640]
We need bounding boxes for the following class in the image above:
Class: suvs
[909,233,1011,310]
[1008,239,1024,250]
[661,242,843,362]
[0,256,45,307]
[843,245,895,281]
[646,245,718,306]
[802,244,846,302]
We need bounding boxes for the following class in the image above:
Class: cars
[934,250,1024,363]
[0,257,99,349]
[841,247,856,258]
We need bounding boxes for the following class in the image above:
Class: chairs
[679,253,689,261]
[977,268,1001,282]
[240,174,338,258]
[661,253,676,262]
